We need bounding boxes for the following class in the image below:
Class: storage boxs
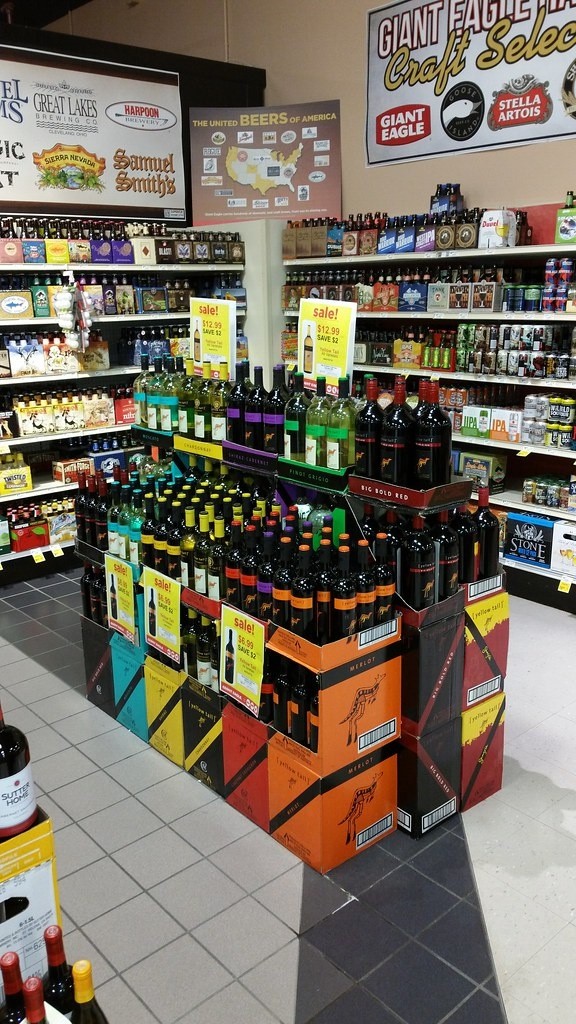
[281,223,500,311]
[0,802,63,1009]
[0,236,175,316]
[0,390,524,876]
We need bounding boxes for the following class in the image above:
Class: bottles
[194,319,200,361]
[565,190,573,208]
[148,588,156,637]
[110,574,117,619]
[225,629,236,684]
[0,184,529,751]
[304,325,313,374]
[0,706,38,837]
[573,195,576,208]
[0,925,111,1024]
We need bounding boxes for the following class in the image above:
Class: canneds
[521,392,576,450]
[454,323,576,381]
[502,256,574,314]
[522,474,570,511]
[438,382,520,433]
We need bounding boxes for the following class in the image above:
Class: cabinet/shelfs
[0,263,246,562]
[284,243,576,584]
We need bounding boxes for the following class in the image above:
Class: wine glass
[478,287,485,307]
[455,288,461,307]
